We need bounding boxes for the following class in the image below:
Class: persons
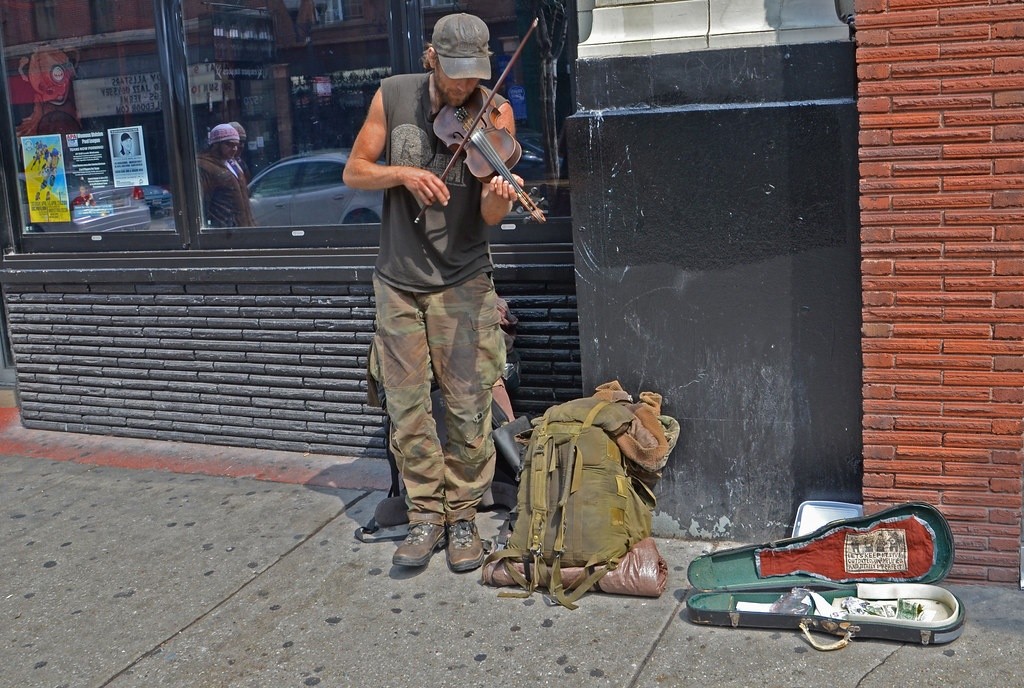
[343,12,524,571]
[197,122,254,226]
[71,181,95,205]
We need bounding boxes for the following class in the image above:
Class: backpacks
[481,397,659,611]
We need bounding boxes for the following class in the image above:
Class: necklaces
[435,90,437,112]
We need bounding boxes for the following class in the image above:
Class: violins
[432,85,546,226]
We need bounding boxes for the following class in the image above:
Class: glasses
[220,141,239,148]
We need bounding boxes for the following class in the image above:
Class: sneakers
[392,523,446,567]
[446,519,485,572]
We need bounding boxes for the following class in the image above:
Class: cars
[17,170,151,234]
[245,150,387,225]
[513,132,565,180]
[138,185,174,218]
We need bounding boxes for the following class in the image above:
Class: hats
[208,124,239,145]
[432,12,492,80]
